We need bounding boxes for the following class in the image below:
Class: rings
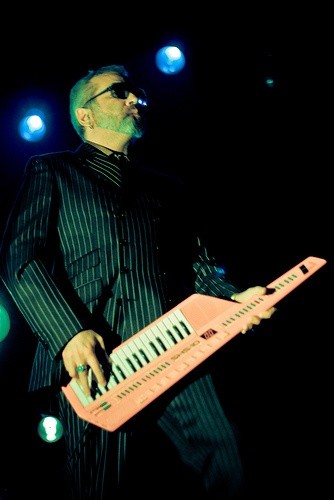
[76,365,88,372]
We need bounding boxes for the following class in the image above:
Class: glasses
[83,79,146,106]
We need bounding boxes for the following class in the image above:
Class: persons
[0,64,275,500]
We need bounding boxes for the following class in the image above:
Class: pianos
[61,252,327,431]
